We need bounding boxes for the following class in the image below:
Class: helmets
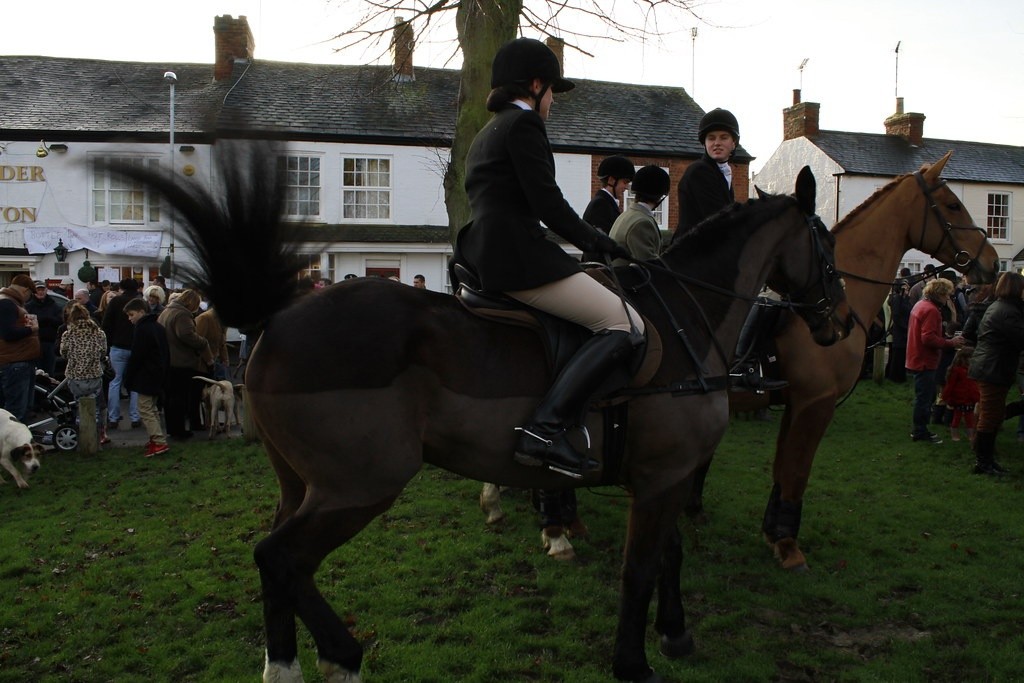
[491,37,576,94]
[698,109,740,146]
[631,166,670,200]
[597,156,636,180]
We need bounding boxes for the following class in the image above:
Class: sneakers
[142,438,170,457]
[910,432,943,444]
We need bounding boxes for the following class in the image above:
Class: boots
[931,406,944,425]
[729,296,791,393]
[945,409,954,432]
[974,432,1011,475]
[512,329,636,473]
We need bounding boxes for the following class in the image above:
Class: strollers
[23,355,116,452]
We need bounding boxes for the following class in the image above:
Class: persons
[609,165,673,263]
[413,275,426,289]
[386,272,401,283]
[886,263,1024,476]
[445,38,647,484]
[319,278,332,288]
[668,108,740,247]
[582,155,636,266]
[0,274,250,458]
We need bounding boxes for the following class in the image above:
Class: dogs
[193,375,235,439]
[0,408,45,488]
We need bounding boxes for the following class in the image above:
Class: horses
[471,149,1001,572]
[71,100,856,683]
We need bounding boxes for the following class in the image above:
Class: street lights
[162,70,177,289]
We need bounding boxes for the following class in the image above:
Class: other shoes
[99,428,111,443]
[131,422,141,428]
[107,420,119,428]
[170,431,193,439]
[968,433,976,441]
[951,432,961,442]
[191,424,207,431]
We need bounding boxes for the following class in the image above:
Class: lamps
[179,146,195,153]
[36,139,68,157]
[53,238,68,262]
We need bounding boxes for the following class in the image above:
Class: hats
[32,280,46,288]
[11,274,38,294]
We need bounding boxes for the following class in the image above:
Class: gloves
[595,235,632,259]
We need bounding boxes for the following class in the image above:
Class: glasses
[29,291,33,297]
[149,294,159,299]
